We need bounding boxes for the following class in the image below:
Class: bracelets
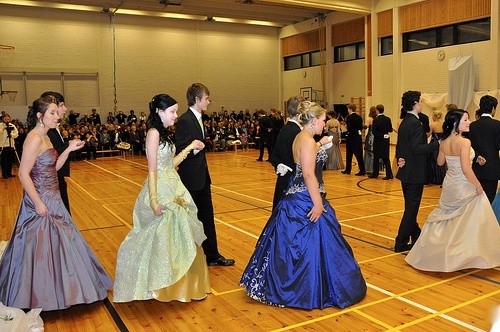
[319,141,322,146]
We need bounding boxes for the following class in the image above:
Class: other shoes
[341,170,350,174]
[368,175,377,178]
[382,177,392,180]
[356,172,365,175]
[395,244,412,252]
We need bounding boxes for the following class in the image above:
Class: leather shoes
[207,256,236,266]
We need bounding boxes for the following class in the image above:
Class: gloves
[322,136,333,149]
[276,163,293,176]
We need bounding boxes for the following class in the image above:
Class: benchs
[233,143,256,150]
[82,150,120,154]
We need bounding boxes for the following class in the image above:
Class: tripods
[0,130,20,164]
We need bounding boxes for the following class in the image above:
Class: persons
[0,96,115,311]
[394,90,500,273]
[317,103,394,181]
[202,106,284,162]
[58,108,151,162]
[110,94,212,303]
[270,96,330,216]
[238,101,367,310]
[0,111,27,178]
[42,92,83,219]
[174,83,236,266]
